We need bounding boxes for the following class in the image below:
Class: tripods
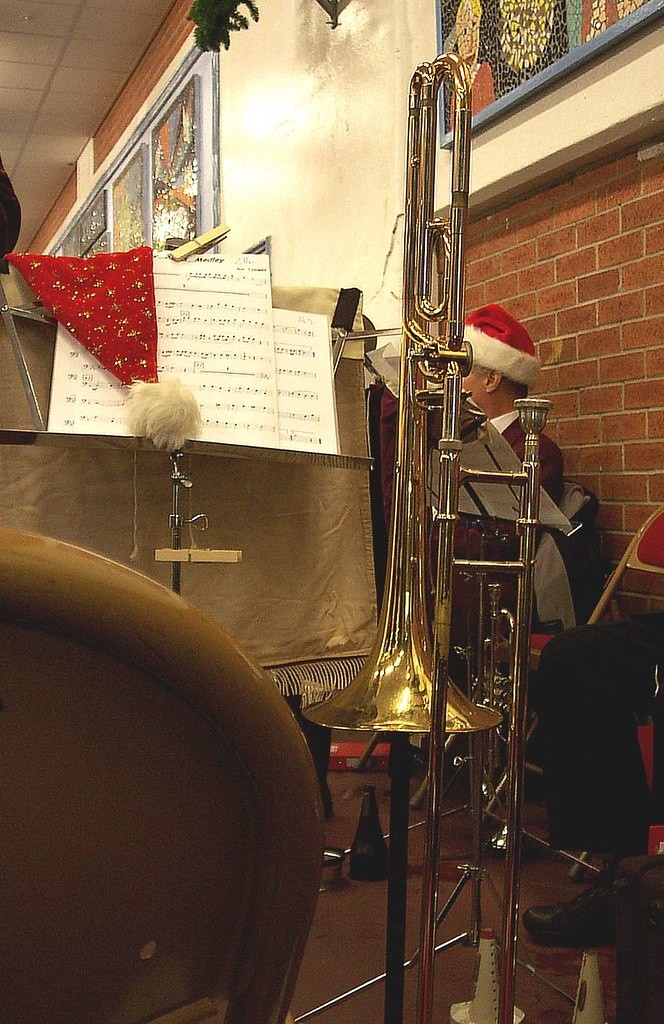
[410,419,585,970]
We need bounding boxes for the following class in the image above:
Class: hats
[463,304,541,387]
[3,246,202,450]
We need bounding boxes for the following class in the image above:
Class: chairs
[480,509,664,878]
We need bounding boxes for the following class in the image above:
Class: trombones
[300,54,555,1024]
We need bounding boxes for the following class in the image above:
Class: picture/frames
[62,74,200,255]
[435,0,664,150]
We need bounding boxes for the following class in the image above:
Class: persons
[522,607,664,950]
[284,303,562,818]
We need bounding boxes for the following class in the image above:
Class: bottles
[350,784,389,882]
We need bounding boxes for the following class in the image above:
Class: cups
[317,845,345,891]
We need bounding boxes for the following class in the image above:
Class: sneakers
[524,881,623,947]
